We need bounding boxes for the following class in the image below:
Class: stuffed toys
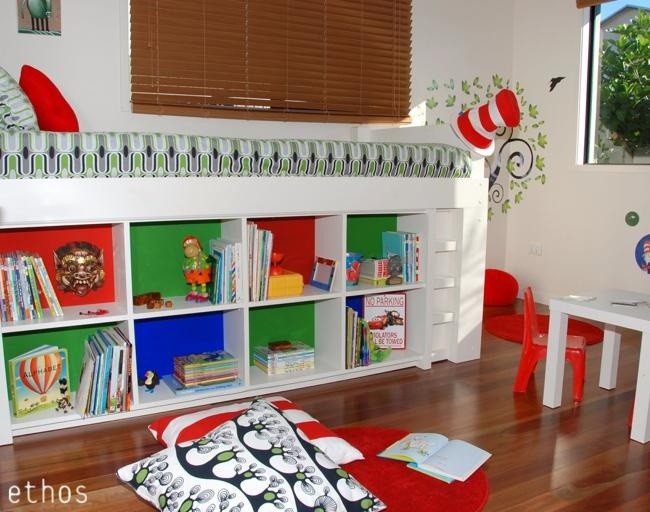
[181,235,211,303]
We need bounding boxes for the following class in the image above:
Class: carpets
[327,424,491,512]
[481,310,607,352]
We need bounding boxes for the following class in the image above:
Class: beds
[0,63,487,176]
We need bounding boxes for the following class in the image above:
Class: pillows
[15,64,81,135]
[108,391,391,512]
[0,65,41,135]
[145,394,368,473]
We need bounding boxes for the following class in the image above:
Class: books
[405,464,455,485]
[381,230,419,284]
[163,350,242,396]
[362,292,407,351]
[376,433,492,483]
[0,249,64,323]
[76,327,134,419]
[7,344,72,417]
[210,220,273,305]
[252,341,315,376]
[345,305,371,368]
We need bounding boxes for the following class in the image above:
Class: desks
[539,285,650,446]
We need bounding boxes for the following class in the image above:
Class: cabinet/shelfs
[1,171,491,452]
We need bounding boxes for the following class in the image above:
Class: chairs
[510,285,590,403]
